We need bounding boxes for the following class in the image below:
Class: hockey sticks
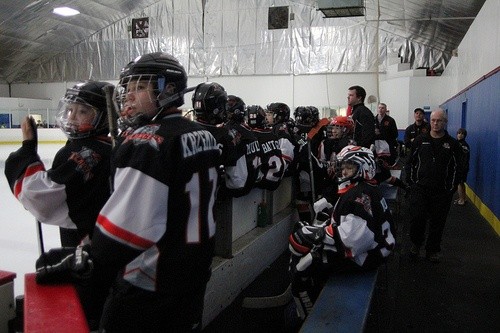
[103,84,118,150]
[31,124,44,254]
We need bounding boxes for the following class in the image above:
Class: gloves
[32,242,97,282]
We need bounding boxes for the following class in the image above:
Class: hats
[413,107,425,115]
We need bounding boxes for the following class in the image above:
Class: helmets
[191,82,227,127]
[326,115,355,142]
[293,106,313,128]
[332,144,377,190]
[226,95,246,126]
[112,51,187,126]
[55,80,121,141]
[245,104,268,130]
[266,102,290,125]
[305,106,320,127]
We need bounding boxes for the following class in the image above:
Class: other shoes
[451,199,468,207]
[410,244,444,264]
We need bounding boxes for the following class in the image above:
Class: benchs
[299,262,380,332]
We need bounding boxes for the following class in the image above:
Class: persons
[453,127,472,205]
[294,106,410,229]
[401,107,466,267]
[245,103,286,191]
[1,119,64,131]
[187,83,248,200]
[404,108,432,194]
[222,95,269,198]
[34,52,223,333]
[282,145,399,327]
[264,103,303,178]
[348,86,376,147]
[7,80,123,251]
[374,101,399,143]
